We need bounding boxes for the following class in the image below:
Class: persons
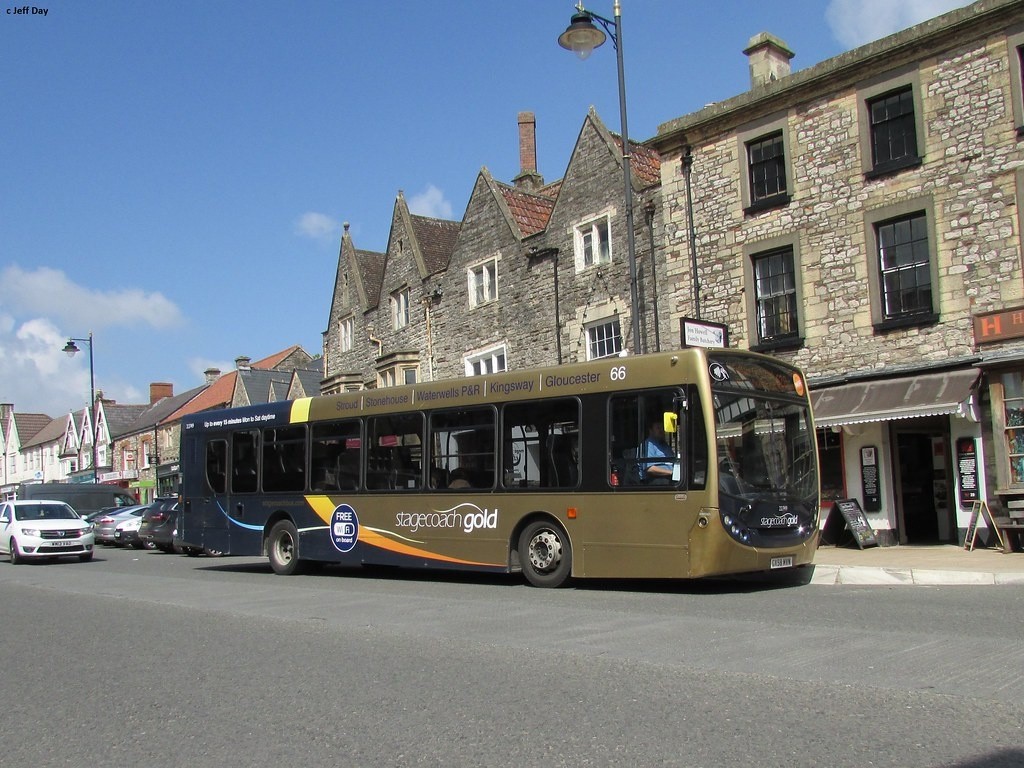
[637,421,677,485]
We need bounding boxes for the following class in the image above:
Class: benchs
[997,500,1024,552]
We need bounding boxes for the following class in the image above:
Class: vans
[17,482,141,518]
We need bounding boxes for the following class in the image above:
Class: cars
[81,504,158,550]
[0,499,96,565]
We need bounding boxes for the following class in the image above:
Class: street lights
[557,0,642,357]
[62,330,98,483]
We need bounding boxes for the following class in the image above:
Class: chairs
[215,437,560,491]
[636,442,650,486]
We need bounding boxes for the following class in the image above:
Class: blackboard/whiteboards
[821,497,877,545]
[964,501,1003,544]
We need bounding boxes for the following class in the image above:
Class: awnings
[716,367,984,437]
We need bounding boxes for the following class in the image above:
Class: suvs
[138,496,225,558]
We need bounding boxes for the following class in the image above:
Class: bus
[176,344,823,589]
